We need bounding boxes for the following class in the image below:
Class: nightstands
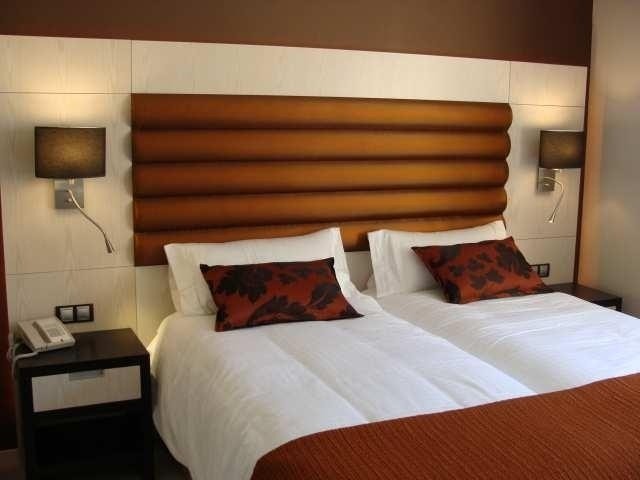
[545,283,623,312]
[12,328,156,480]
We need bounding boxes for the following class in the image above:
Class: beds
[130,92,640,480]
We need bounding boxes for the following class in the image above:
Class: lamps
[33,123,116,256]
[536,129,587,192]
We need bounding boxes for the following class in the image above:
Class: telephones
[16,316,75,352]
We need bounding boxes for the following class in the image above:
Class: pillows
[366,219,508,303]
[200,258,363,333]
[162,226,365,324]
[411,236,551,305]
